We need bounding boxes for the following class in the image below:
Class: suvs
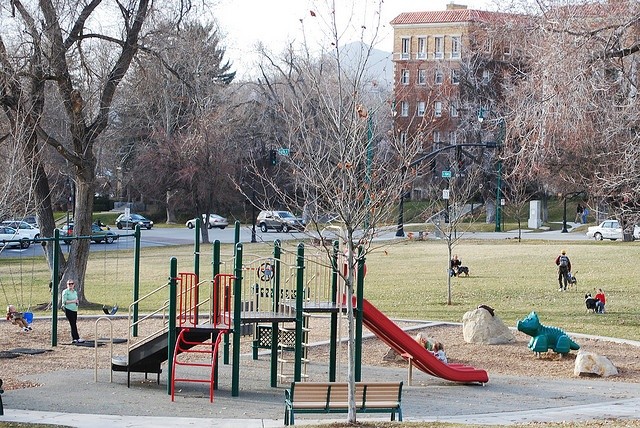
[256,210,306,233]
[2,221,41,243]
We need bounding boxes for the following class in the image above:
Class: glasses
[67,283,74,285]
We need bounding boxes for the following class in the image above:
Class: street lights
[478,107,503,231]
[364,96,397,238]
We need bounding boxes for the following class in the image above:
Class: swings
[101,235,120,315]
[20,238,36,324]
[0,241,23,325]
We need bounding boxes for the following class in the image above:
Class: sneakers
[78,339,85,343]
[21,327,29,332]
[27,326,32,330]
[72,340,77,343]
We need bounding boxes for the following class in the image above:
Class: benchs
[284,381,404,425]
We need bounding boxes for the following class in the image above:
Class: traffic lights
[269,149,276,165]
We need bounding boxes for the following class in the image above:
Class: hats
[561,249,566,253]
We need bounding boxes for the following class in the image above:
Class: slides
[110,320,227,374]
[342,292,489,387]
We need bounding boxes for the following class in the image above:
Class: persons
[573,203,583,224]
[555,249,571,291]
[426,343,447,366]
[449,254,461,277]
[594,288,606,314]
[7,304,32,331]
[61,278,85,342]
[415,332,434,351]
[583,206,588,224]
[580,205,584,223]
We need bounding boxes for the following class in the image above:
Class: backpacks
[559,255,568,267]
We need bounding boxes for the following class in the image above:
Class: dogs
[452,264,470,277]
[568,270,579,290]
[584,293,597,315]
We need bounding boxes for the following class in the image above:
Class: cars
[63,223,118,244]
[186,213,229,229]
[0,225,30,249]
[586,219,640,241]
[117,213,153,230]
